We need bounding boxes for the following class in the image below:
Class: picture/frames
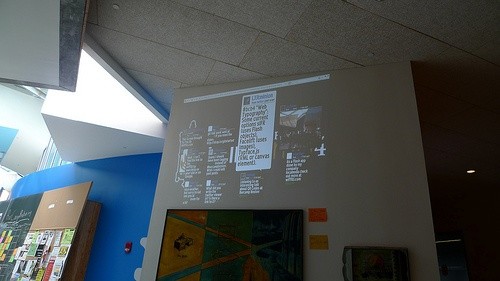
[342,245,411,281]
[156,208,304,280]
